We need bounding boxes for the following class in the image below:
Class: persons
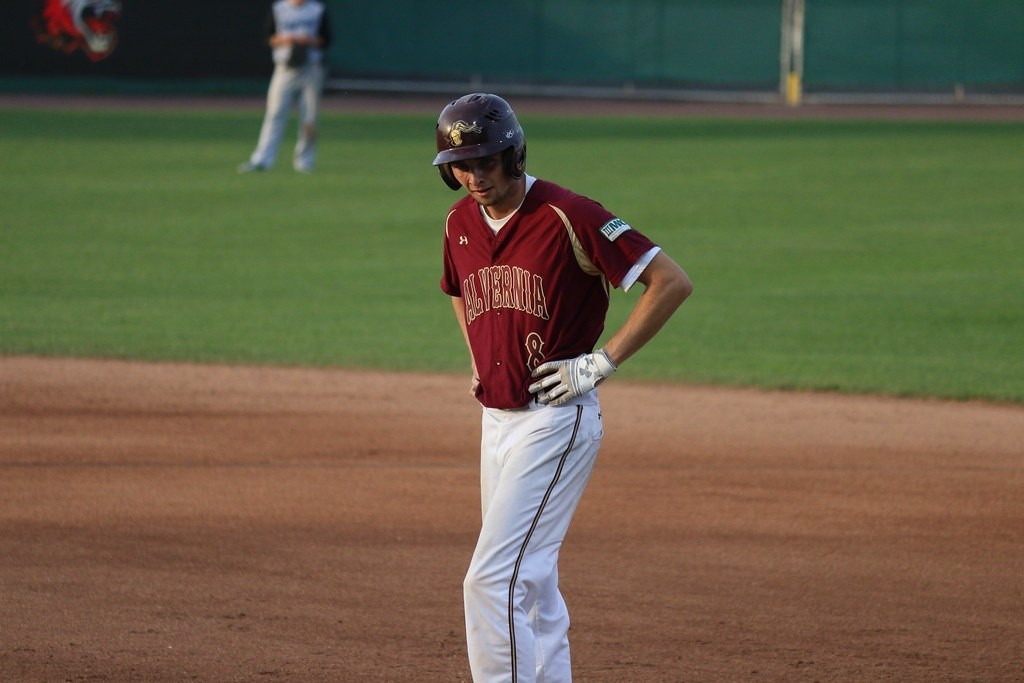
[232,0,337,176]
[431,91,696,683]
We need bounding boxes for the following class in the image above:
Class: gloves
[527,349,617,406]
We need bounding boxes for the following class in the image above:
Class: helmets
[432,93,527,191]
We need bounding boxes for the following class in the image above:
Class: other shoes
[294,159,315,174]
[237,161,271,173]
